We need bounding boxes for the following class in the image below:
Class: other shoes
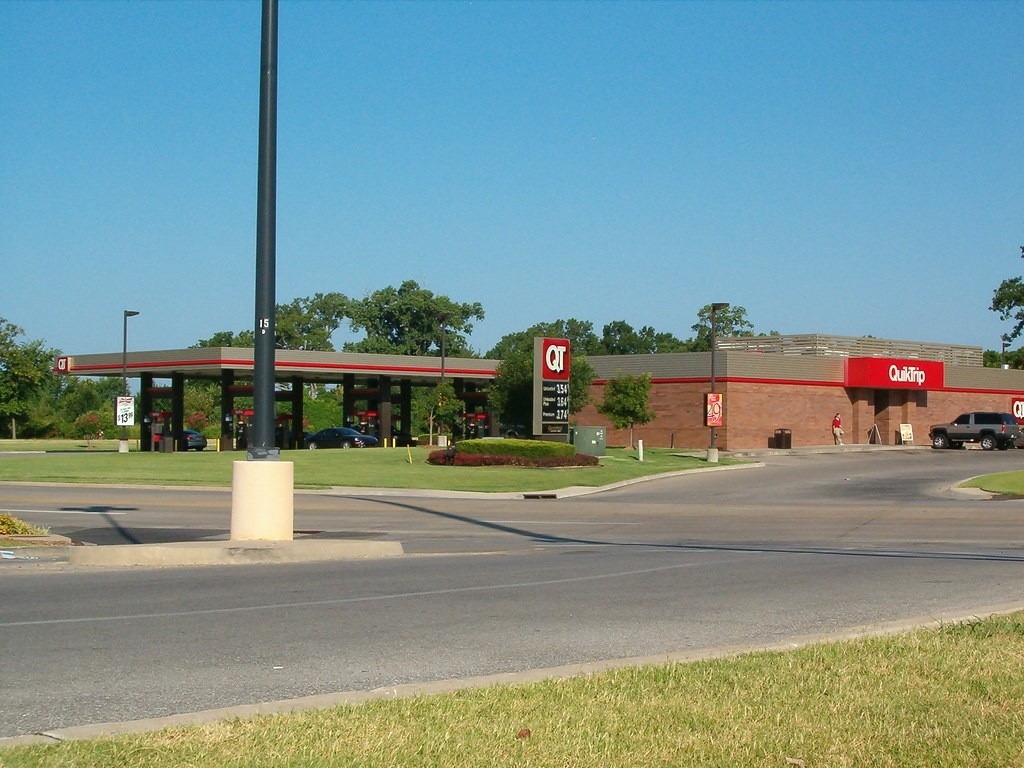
[839,442,844,445]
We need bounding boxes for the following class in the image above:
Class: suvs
[929,411,1020,452]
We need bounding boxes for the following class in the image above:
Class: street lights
[119,310,140,453]
[438,311,454,447]
[705,302,731,464]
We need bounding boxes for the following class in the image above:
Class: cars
[181,428,207,451]
[304,426,378,450]
[372,424,417,447]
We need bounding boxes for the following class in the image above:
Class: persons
[833,413,844,445]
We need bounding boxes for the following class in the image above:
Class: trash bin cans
[159,437,173,453]
[774,428,791,449]
